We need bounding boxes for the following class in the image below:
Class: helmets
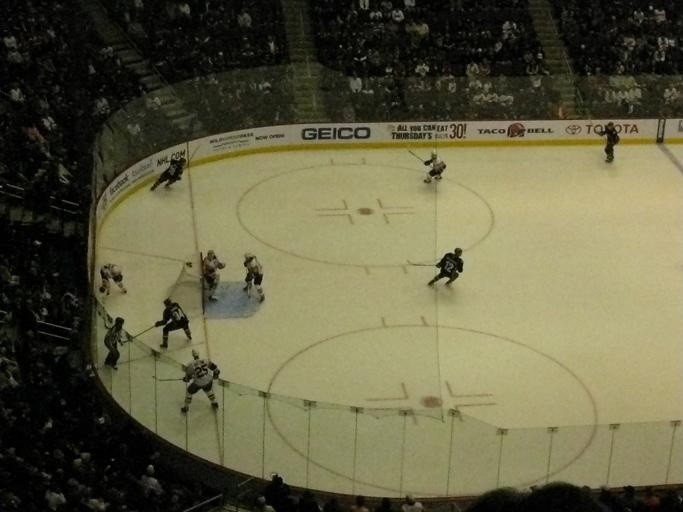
[207,250,215,258]
[455,248,462,256]
[607,121,615,131]
[179,158,186,167]
[164,298,171,306]
[431,152,438,160]
[244,250,254,260]
[111,266,120,276]
[116,318,125,326]
[192,348,199,358]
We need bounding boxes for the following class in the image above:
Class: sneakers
[181,407,189,412]
[243,285,252,290]
[112,364,118,370]
[121,288,127,293]
[605,155,615,163]
[424,176,442,183]
[259,295,265,302]
[160,343,168,347]
[212,403,218,408]
[189,336,193,340]
[208,297,218,302]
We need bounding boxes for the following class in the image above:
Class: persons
[144,442,682,510]
[150,158,188,190]
[102,3,301,134]
[426,248,464,287]
[307,3,567,123]
[550,3,681,120]
[182,349,221,413]
[3,156,139,510]
[202,249,226,302]
[4,2,149,152]
[242,252,266,302]
[598,121,620,163]
[423,151,446,184]
[155,297,192,349]
